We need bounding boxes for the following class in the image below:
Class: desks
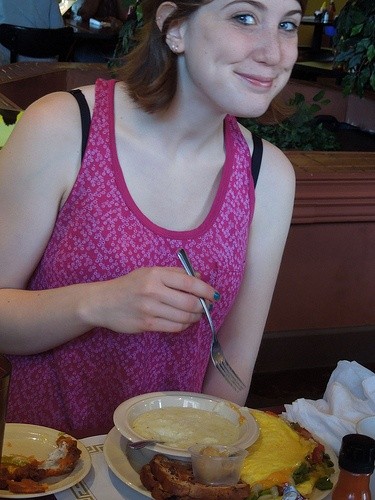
[300,15,341,60]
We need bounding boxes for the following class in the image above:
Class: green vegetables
[248,421,334,500]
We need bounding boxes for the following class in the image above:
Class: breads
[0,436,82,494]
[139,453,250,500]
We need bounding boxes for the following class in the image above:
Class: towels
[283,359,375,457]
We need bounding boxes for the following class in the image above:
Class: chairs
[0,23,120,65]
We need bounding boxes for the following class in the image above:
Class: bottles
[320,1,329,21]
[333,433,375,500]
[329,2,336,22]
[66,7,71,20]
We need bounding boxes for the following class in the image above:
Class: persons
[0,0,308,439]
[311,1,340,49]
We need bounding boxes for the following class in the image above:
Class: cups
[0,354,11,463]
[190,442,249,486]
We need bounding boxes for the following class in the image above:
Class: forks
[176,246,247,392]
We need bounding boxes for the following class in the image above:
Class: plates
[103,408,341,500]
[0,421,92,500]
[113,391,261,463]
